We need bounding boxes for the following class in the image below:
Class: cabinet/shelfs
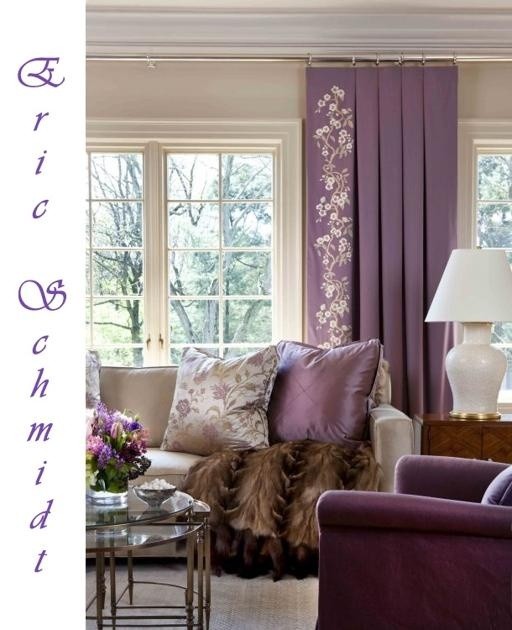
[415,414,512,464]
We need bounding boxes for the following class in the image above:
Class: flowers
[83,402,151,492]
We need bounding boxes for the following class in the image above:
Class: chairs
[317,455,512,630]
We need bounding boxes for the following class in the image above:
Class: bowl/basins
[133,488,176,505]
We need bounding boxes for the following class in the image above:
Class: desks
[85,487,211,629]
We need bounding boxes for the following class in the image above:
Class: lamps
[424,248,512,420]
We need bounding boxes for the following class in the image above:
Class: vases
[89,471,127,509]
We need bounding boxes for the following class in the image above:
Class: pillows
[161,346,280,458]
[267,339,382,445]
[481,464,512,506]
[84,350,101,414]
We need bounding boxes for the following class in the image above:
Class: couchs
[84,361,414,563]
